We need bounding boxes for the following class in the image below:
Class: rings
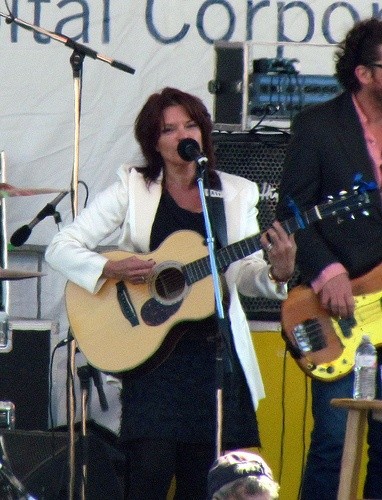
[265,242,273,252]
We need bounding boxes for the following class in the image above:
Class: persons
[43,88,298,500]
[275,14,381,500]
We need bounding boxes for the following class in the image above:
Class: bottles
[353,335,377,401]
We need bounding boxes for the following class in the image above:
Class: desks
[330,398,382,500]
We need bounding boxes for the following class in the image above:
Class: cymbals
[0,267,47,281]
[0,183,74,198]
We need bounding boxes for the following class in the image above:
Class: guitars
[280,263,382,382]
[64,182,382,381]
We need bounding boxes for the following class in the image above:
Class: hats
[207,451,274,500]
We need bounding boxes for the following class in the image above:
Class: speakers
[207,128,289,321]
[0,430,128,500]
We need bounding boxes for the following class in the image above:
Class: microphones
[177,138,208,169]
[9,187,72,247]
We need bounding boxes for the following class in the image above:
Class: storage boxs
[0,314,60,430]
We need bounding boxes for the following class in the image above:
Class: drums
[0,402,16,431]
